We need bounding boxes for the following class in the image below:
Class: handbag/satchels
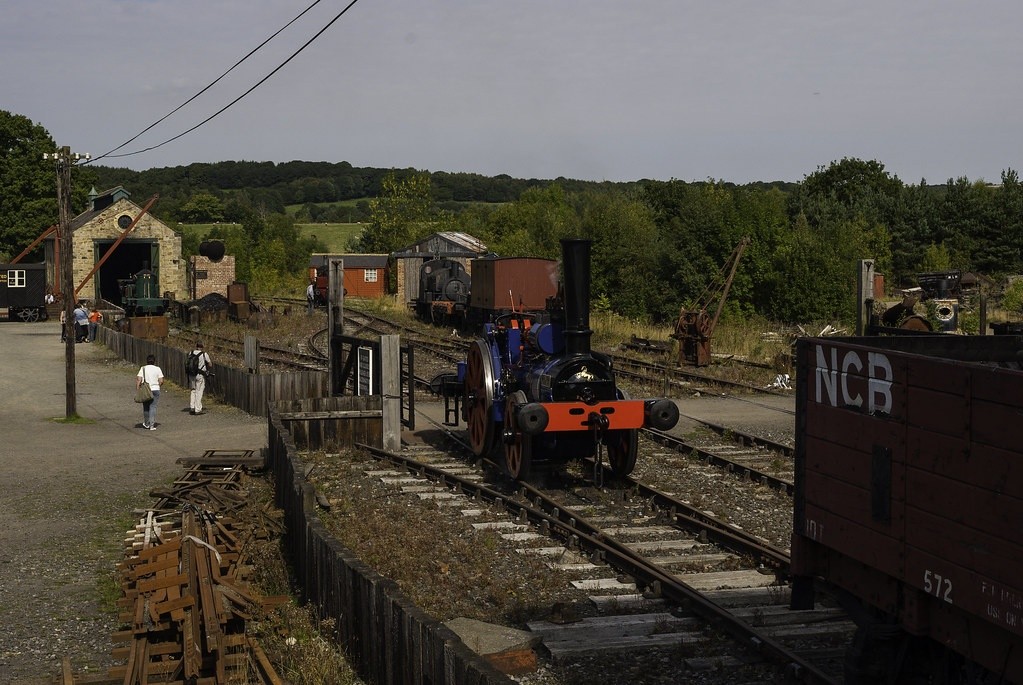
[134,366,155,403]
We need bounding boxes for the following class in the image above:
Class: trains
[432,236,681,484]
[411,243,559,333]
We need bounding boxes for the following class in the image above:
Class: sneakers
[142,423,149,429]
[149,427,157,431]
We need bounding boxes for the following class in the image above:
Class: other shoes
[190,410,195,415]
[195,412,207,415]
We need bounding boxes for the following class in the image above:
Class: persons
[60,307,67,343]
[188,342,212,415]
[45,293,54,304]
[307,282,315,308]
[74,300,102,344]
[136,354,164,430]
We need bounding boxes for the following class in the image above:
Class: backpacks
[184,349,207,377]
